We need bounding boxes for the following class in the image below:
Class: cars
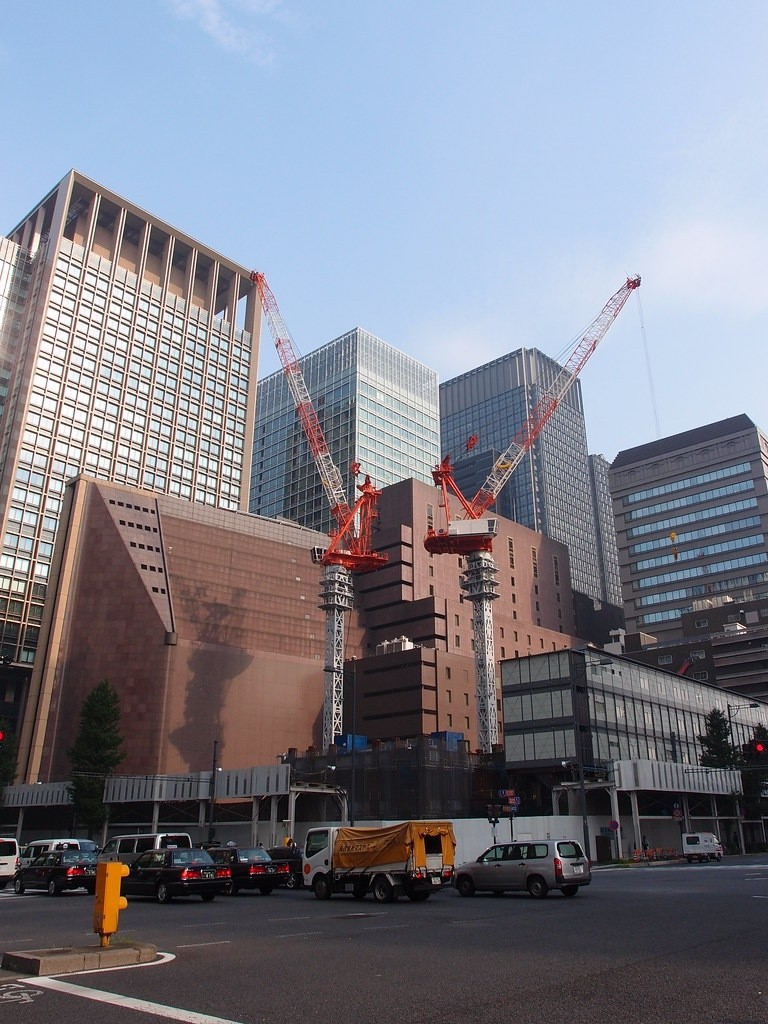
[120,847,234,904]
[12,850,109,895]
[266,846,303,888]
[206,847,290,897]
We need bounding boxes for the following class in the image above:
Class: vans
[0,837,19,889]
[98,833,192,869]
[19,838,80,868]
[77,838,98,850]
[454,839,591,897]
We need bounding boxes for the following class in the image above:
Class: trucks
[682,832,723,863]
[303,819,456,903]
